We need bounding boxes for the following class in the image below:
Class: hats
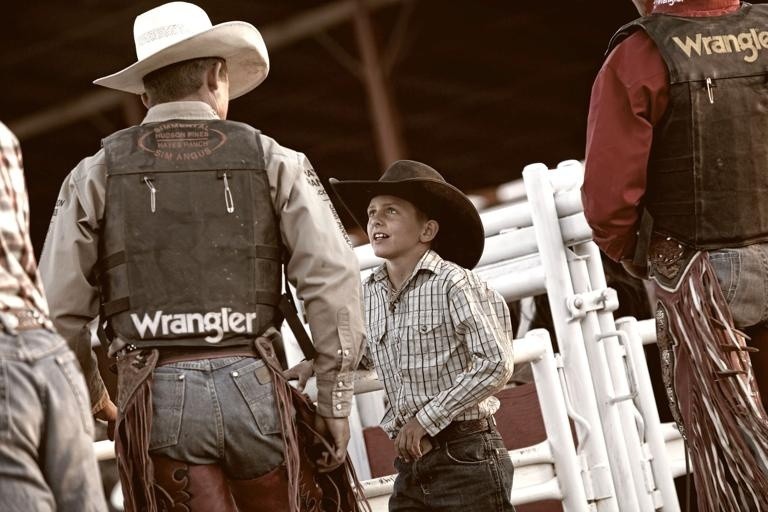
[90,0,274,102]
[326,158,486,272]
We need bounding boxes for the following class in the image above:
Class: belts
[423,414,497,445]
[0,306,45,333]
[144,347,259,368]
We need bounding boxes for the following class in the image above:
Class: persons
[35,1,368,512]
[579,0,768,512]
[0,121,109,512]
[283,160,517,512]
